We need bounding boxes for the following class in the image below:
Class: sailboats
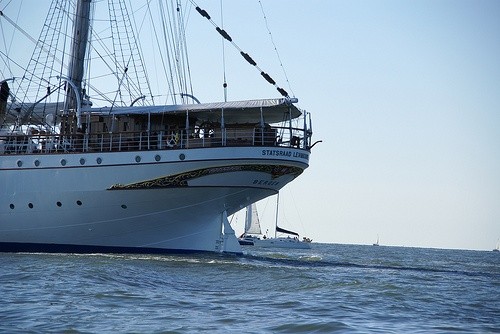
[493,240,499,251]
[373,238,379,246]
[0,0,322,257]
[230,191,312,249]
